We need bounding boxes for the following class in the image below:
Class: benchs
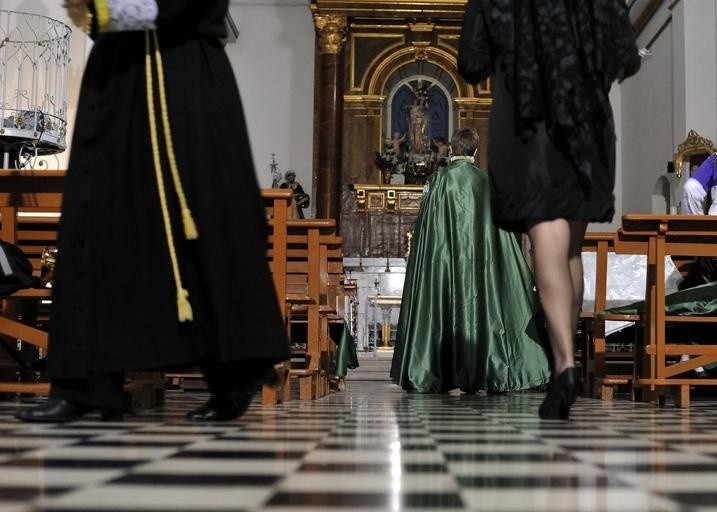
[578,215,717,406]
[261,186,350,407]
[0,168,204,409]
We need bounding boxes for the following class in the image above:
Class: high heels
[539,367,582,421]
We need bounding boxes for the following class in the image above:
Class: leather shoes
[186,366,280,422]
[15,388,124,422]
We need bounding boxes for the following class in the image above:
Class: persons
[430,135,447,157]
[389,131,407,156]
[16,0,294,424]
[281,170,310,219]
[679,152,716,216]
[457,1,642,420]
[388,126,555,398]
[409,96,429,155]
